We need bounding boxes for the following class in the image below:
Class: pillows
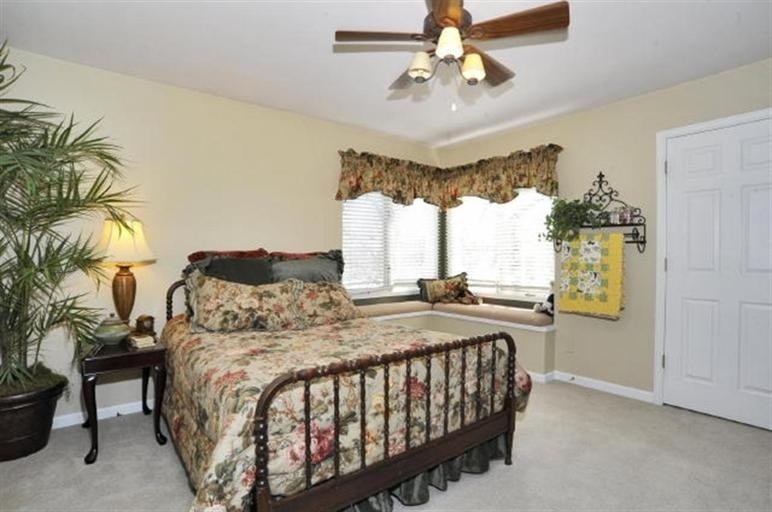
[417,271,468,305]
[179,248,367,332]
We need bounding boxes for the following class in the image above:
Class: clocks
[136,314,157,342]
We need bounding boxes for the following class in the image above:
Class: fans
[334,0,569,112]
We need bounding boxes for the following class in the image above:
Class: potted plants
[0,33,149,464]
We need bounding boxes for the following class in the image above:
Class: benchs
[432,301,555,383]
[357,299,432,332]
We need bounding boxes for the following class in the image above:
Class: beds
[155,278,517,511]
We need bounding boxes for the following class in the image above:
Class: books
[126,330,157,349]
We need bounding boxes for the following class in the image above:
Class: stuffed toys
[531,280,555,318]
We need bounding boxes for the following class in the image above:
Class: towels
[558,232,626,321]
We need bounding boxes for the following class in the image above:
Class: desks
[77,331,168,464]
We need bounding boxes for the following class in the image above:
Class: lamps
[408,25,487,111]
[88,213,157,336]
